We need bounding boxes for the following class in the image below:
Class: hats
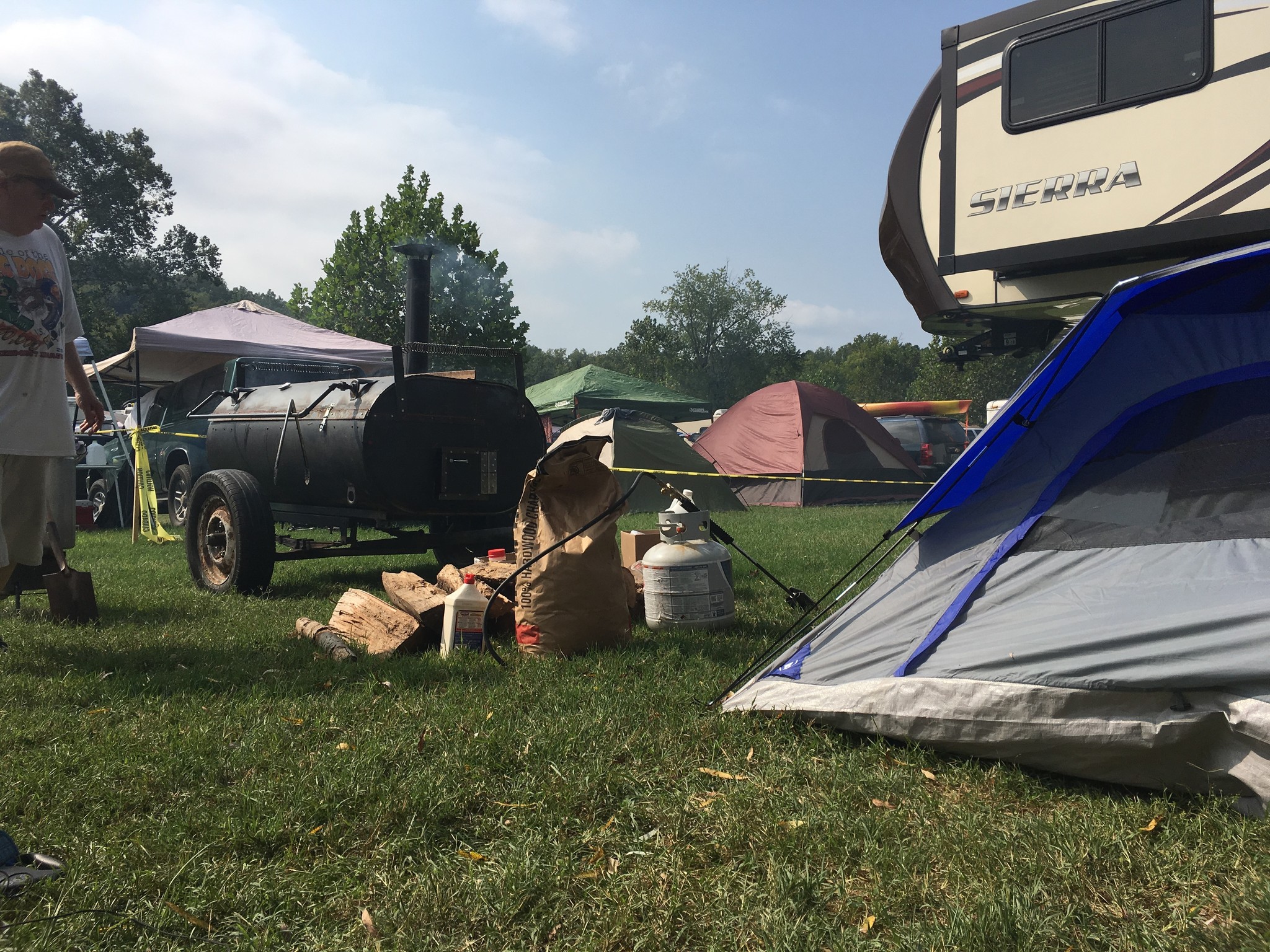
[0,141,77,200]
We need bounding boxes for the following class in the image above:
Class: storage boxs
[620,530,661,595]
[76,500,96,529]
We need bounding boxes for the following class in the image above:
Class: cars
[67,396,124,498]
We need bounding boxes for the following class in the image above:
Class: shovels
[44,522,102,628]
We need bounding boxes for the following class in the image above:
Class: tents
[707,240,1270,822]
[691,380,930,508]
[72,337,155,528]
[547,408,752,518]
[525,364,714,425]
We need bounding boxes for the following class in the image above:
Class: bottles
[86,440,106,466]
[440,574,488,661]
[488,549,507,562]
[663,490,694,532]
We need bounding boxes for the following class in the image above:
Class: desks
[76,464,125,530]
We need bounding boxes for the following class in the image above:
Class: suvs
[86,356,369,529]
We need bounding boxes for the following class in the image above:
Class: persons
[0,141,105,651]
[72,300,405,545]
